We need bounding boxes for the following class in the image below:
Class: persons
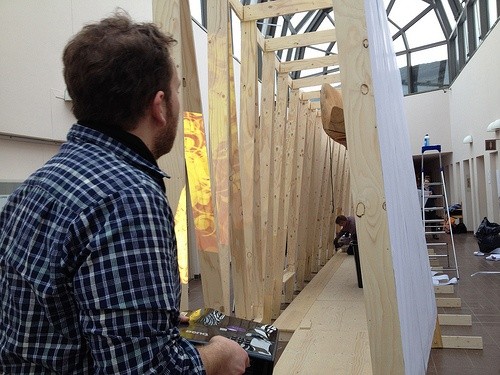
[332,214,358,248]
[419,177,440,239]
[0,18,254,375]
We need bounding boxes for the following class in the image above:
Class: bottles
[423,133,430,147]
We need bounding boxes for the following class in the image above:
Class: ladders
[421,145,460,279]
